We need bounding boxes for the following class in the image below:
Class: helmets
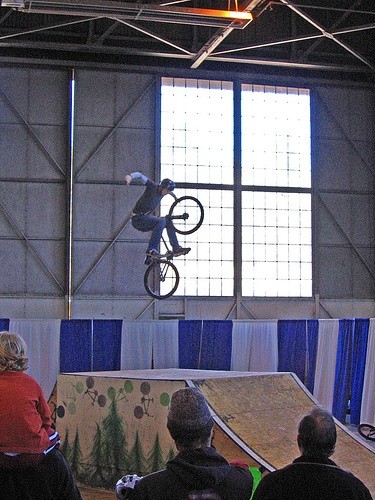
[160,178,174,194]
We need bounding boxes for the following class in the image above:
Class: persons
[0,332,61,468]
[124,171,191,264]
[0,448,84,500]
[128,387,254,500]
[252,409,373,500]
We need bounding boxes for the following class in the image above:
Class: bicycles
[358,423,375,442]
[143,196,203,299]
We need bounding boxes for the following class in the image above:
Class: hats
[167,388,217,445]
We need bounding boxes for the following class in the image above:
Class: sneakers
[144,248,165,258]
[172,245,195,256]
